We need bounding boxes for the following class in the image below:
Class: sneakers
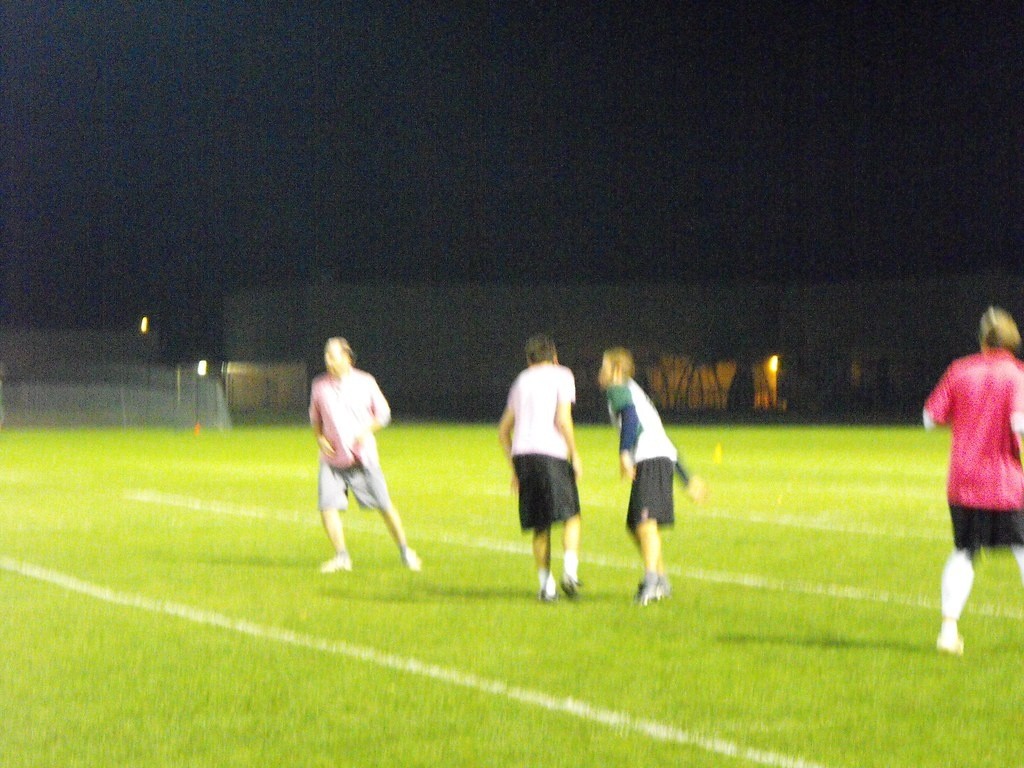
[558,572,585,602]
[631,581,672,608]
[401,548,421,571]
[537,589,563,603]
[321,558,352,573]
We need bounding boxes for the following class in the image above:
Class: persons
[308,337,421,573]
[498,335,581,602]
[922,309,1024,656]
[598,346,698,605]
